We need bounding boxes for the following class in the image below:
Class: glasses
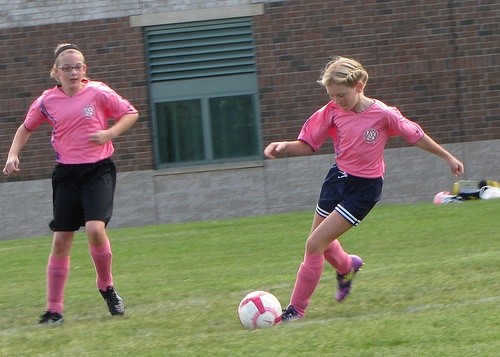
[58,65,83,72]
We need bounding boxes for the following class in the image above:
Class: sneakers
[336,255,363,302]
[282,305,302,321]
[38,311,65,324]
[99,285,125,315]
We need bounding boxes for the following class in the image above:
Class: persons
[264,55,463,326]
[3,43,139,325]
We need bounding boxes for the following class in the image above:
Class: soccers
[238,291,282,331]
